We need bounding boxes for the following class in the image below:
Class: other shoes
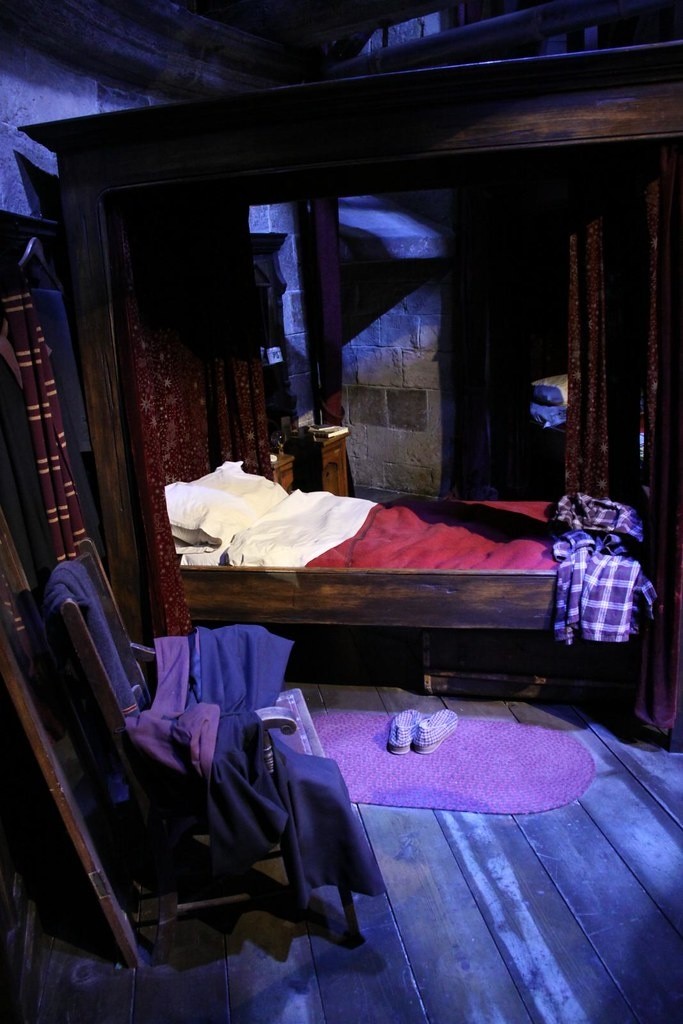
[410,709,457,754]
[388,708,426,754]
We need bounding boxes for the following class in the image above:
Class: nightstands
[285,431,351,497]
[270,453,300,494]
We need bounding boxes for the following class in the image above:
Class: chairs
[55,537,365,968]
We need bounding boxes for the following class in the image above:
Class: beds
[15,38,683,753]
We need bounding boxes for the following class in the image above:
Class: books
[308,424,349,438]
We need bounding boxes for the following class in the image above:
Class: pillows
[165,460,289,546]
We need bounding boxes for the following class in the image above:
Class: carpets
[311,712,596,815]
